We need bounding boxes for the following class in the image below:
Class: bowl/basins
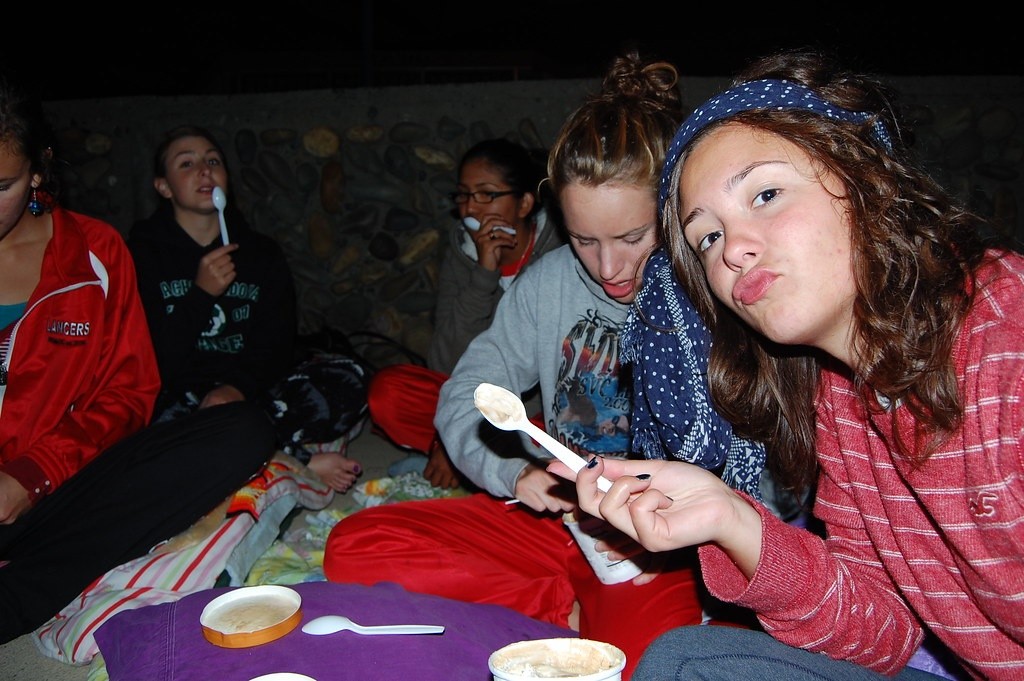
[487,638,627,681]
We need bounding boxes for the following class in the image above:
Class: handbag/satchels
[295,327,365,368]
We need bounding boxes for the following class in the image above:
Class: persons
[324,50,752,681]
[545,44,1024,681]
[366,138,570,489]
[0,73,277,644]
[125,123,362,493]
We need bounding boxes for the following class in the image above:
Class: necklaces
[515,232,532,276]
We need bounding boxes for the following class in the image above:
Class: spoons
[300,614,445,636]
[213,184,230,249]
[464,217,518,235]
[472,382,633,503]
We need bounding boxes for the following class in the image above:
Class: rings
[488,230,496,240]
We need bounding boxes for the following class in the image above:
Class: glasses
[447,186,530,205]
